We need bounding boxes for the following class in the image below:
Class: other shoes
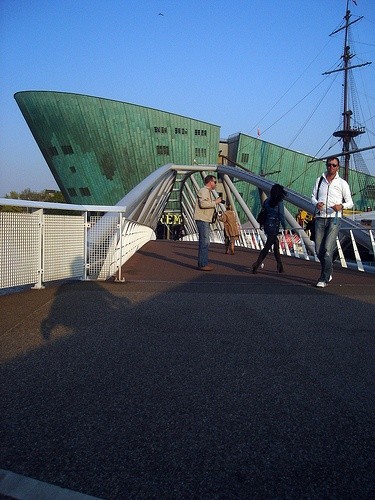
[199,267,213,271]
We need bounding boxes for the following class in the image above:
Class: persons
[310,156,356,288]
[249,183,287,273]
[194,175,223,271]
[218,207,241,255]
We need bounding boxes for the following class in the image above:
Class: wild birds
[257,128,261,136]
[157,13,165,16]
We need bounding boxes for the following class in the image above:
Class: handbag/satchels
[212,212,217,223]
[307,218,315,235]
[256,199,268,225]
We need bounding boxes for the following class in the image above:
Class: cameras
[218,197,225,204]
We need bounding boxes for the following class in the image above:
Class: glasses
[211,180,216,184]
[325,163,338,167]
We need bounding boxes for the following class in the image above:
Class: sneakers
[316,275,332,288]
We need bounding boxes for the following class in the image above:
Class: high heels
[277,261,283,273]
[225,248,229,254]
[231,246,235,255]
[249,264,259,274]
